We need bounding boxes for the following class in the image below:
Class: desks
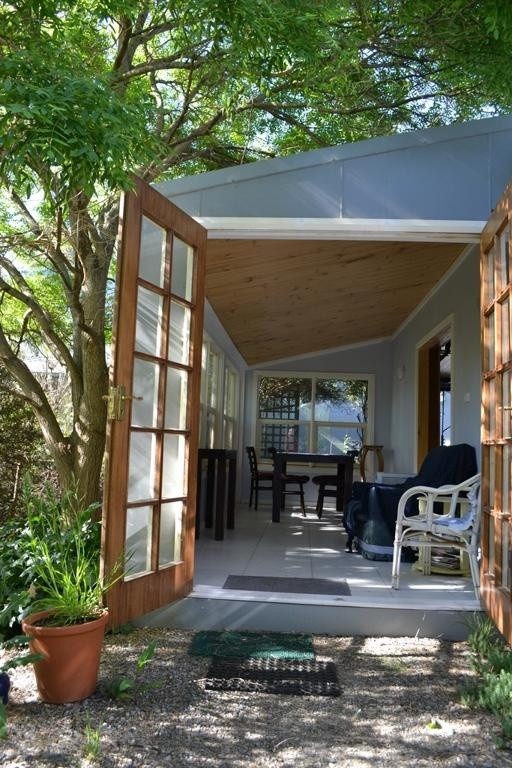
[412,496,473,578]
[376,472,415,486]
[358,446,384,482]
[196,449,237,541]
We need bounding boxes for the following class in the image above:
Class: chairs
[245,446,273,510]
[268,447,309,517]
[391,472,482,600]
[343,444,478,562]
[312,450,358,519]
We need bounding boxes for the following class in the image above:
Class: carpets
[205,655,341,697]
[187,630,315,661]
[222,575,352,597]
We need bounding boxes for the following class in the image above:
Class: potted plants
[11,468,136,703]
